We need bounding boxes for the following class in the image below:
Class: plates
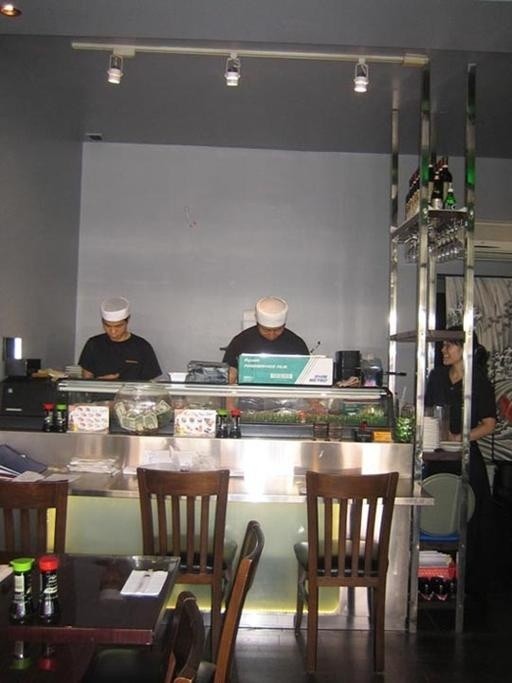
[420,471,479,538]
[422,416,461,453]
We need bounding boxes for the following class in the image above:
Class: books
[0,445,47,477]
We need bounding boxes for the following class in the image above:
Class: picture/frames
[432,271,512,465]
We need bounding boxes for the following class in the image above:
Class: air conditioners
[456,216,512,262]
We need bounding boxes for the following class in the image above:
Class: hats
[255,295,289,327]
[101,296,130,321]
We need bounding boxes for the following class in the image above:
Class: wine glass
[405,219,464,263]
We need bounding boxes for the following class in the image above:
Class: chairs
[134,466,240,663]
[159,591,206,681]
[293,470,401,676]
[0,631,96,682]
[91,520,264,681]
[1,480,72,553]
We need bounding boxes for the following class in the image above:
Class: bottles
[36,642,57,673]
[216,408,242,437]
[37,554,59,601]
[404,154,455,226]
[416,574,456,604]
[10,639,29,672]
[9,600,30,624]
[11,557,32,599]
[40,601,56,624]
[40,402,68,432]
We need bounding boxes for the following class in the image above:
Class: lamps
[67,34,431,96]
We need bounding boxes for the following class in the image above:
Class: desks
[0,553,183,682]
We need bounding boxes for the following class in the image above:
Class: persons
[92,558,130,607]
[78,297,163,404]
[222,296,329,414]
[425,325,497,501]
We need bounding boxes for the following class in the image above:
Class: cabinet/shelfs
[388,69,480,638]
[49,377,398,440]
